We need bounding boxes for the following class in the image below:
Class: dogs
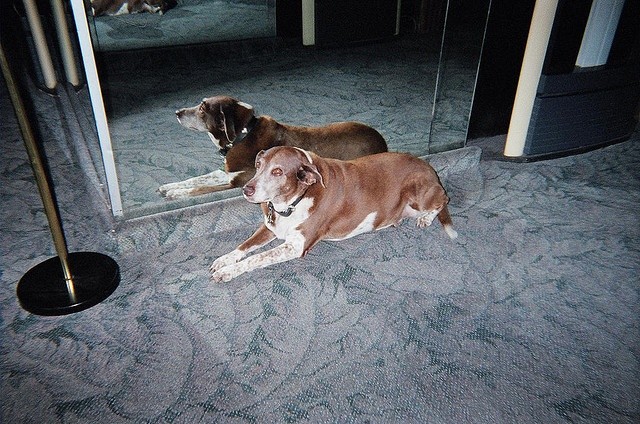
[208,146,459,282]
[155,95,387,200]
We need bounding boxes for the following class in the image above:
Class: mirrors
[70,0,493,224]
